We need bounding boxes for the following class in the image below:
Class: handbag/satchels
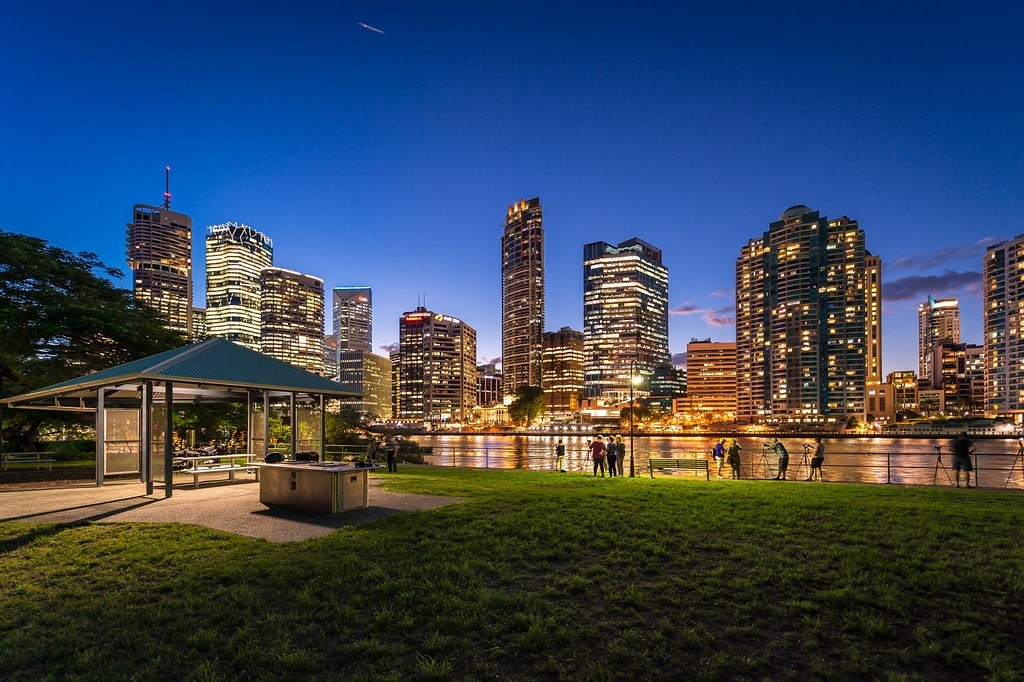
[726,455,733,464]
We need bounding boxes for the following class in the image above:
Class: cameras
[587,440,591,442]
[802,444,807,448]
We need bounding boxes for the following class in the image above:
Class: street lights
[627,359,642,478]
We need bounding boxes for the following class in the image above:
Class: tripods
[579,443,593,478]
[794,445,814,481]
[753,449,774,480]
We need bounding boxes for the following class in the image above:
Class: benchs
[649,459,711,482]
[0,452,56,471]
[161,465,260,489]
[268,446,292,461]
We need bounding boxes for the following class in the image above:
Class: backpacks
[710,448,716,460]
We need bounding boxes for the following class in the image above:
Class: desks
[243,462,385,518]
[182,454,257,488]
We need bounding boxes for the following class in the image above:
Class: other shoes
[804,479,812,481]
[780,478,786,480]
[772,478,780,480]
[717,476,723,479]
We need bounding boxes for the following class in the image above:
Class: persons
[714,439,742,480]
[770,438,789,480]
[367,436,398,472]
[952,432,978,488]
[589,435,626,478]
[555,440,565,472]
[804,438,825,482]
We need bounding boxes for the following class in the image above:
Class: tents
[0,336,371,497]
